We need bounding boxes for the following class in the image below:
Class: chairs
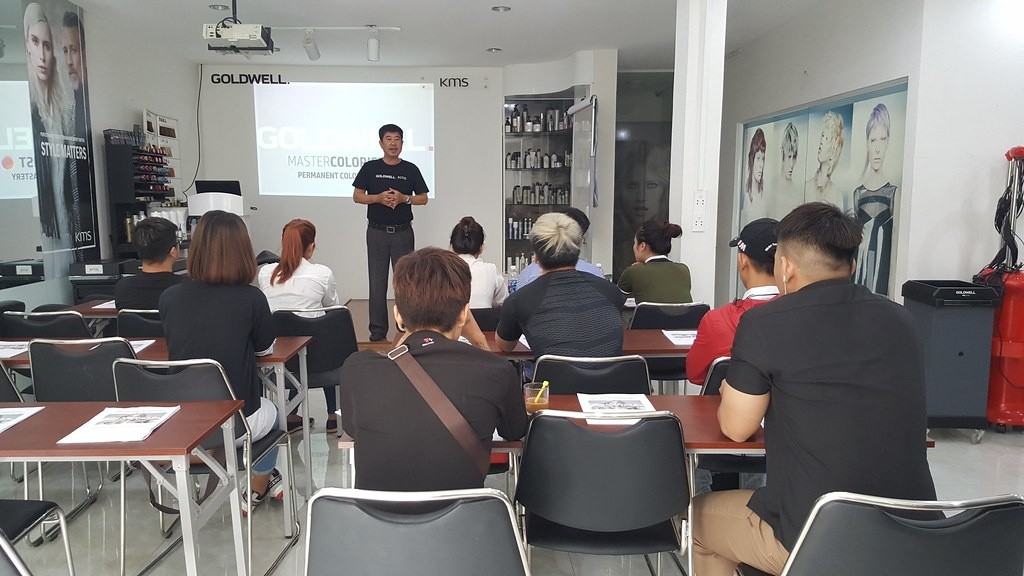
[0,300,1024,576]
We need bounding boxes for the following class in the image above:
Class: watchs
[406,194,412,205]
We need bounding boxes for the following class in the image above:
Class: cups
[523,382,549,416]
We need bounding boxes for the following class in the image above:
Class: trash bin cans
[901,280,1003,444]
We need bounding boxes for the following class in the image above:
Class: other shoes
[369,334,385,341]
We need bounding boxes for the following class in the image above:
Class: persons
[613,137,669,276]
[851,104,898,298]
[157,210,283,517]
[115,217,190,321]
[773,122,802,218]
[339,247,530,514]
[804,110,845,214]
[617,217,693,329]
[740,128,768,227]
[692,200,946,576]
[515,206,604,291]
[685,216,780,492]
[23,2,103,281]
[495,212,624,386]
[352,124,430,343]
[257,219,337,433]
[445,216,510,310]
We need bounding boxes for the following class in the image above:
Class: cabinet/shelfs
[103,129,191,257]
[502,96,575,278]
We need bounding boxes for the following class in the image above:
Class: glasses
[168,237,182,253]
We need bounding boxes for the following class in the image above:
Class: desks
[0,336,314,539]
[390,328,698,358]
[58,298,352,337]
[0,400,246,576]
[337,394,934,490]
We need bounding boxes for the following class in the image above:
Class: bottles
[508,265,518,295]
[595,264,604,277]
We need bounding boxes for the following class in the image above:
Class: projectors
[203,24,275,55]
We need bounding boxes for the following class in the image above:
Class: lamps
[367,30,380,61]
[302,30,320,61]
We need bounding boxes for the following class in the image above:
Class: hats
[729,218,779,263]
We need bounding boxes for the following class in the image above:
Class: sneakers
[241,486,269,516]
[326,420,337,432]
[286,417,315,433]
[267,468,283,501]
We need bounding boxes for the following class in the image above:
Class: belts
[369,221,410,234]
